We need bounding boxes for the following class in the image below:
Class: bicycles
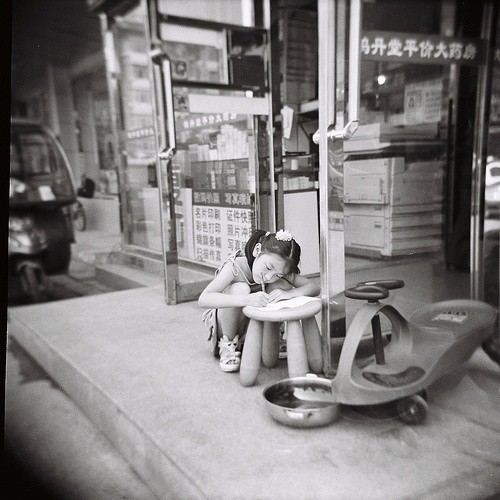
[70,200,86,232]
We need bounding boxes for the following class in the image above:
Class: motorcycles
[10,117,88,302]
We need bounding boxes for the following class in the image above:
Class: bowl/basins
[261,377,341,429]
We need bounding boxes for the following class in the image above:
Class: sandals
[217,335,240,372]
[279,338,288,360]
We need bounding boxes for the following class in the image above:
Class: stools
[240,296,325,387]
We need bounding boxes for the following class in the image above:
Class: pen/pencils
[260,272,266,293]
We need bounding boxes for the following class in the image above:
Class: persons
[198,229,322,373]
